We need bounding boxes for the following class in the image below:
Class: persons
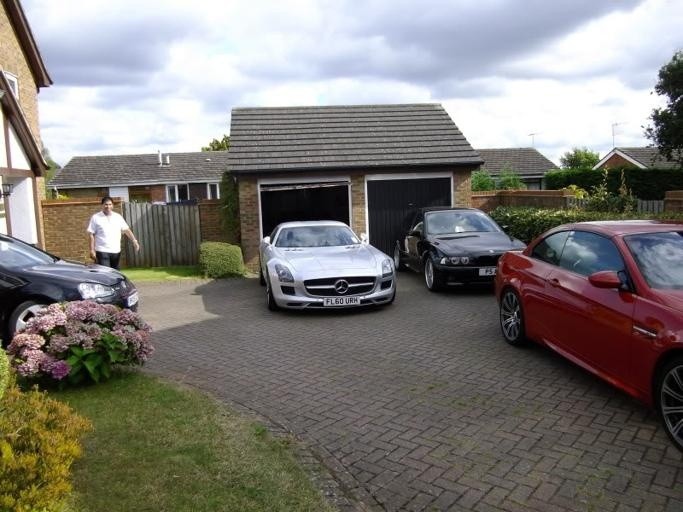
[87,197,139,269]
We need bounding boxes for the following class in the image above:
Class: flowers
[5,299,154,391]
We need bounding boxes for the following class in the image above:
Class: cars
[495,219,683,450]
[394,207,527,292]
[1,234,140,340]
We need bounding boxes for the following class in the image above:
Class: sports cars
[259,220,396,311]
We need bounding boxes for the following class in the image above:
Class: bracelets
[131,239,138,245]
